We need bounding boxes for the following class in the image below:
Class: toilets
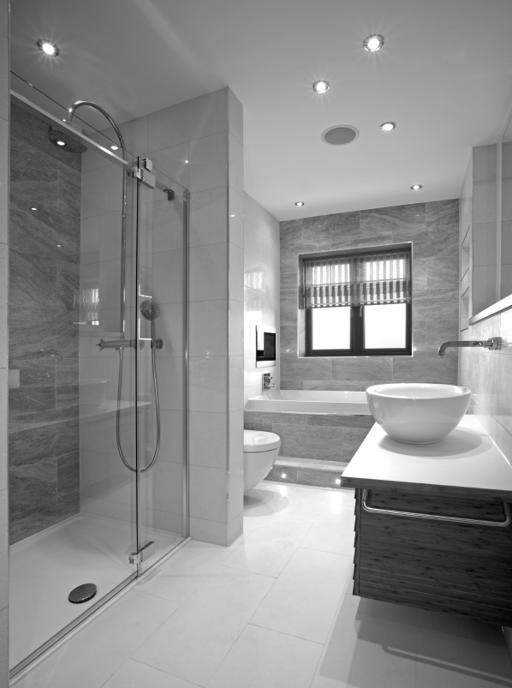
[242,430,280,497]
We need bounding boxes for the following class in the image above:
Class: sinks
[366,383,470,444]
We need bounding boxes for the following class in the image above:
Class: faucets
[438,337,501,354]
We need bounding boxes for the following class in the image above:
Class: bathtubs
[247,389,372,415]
